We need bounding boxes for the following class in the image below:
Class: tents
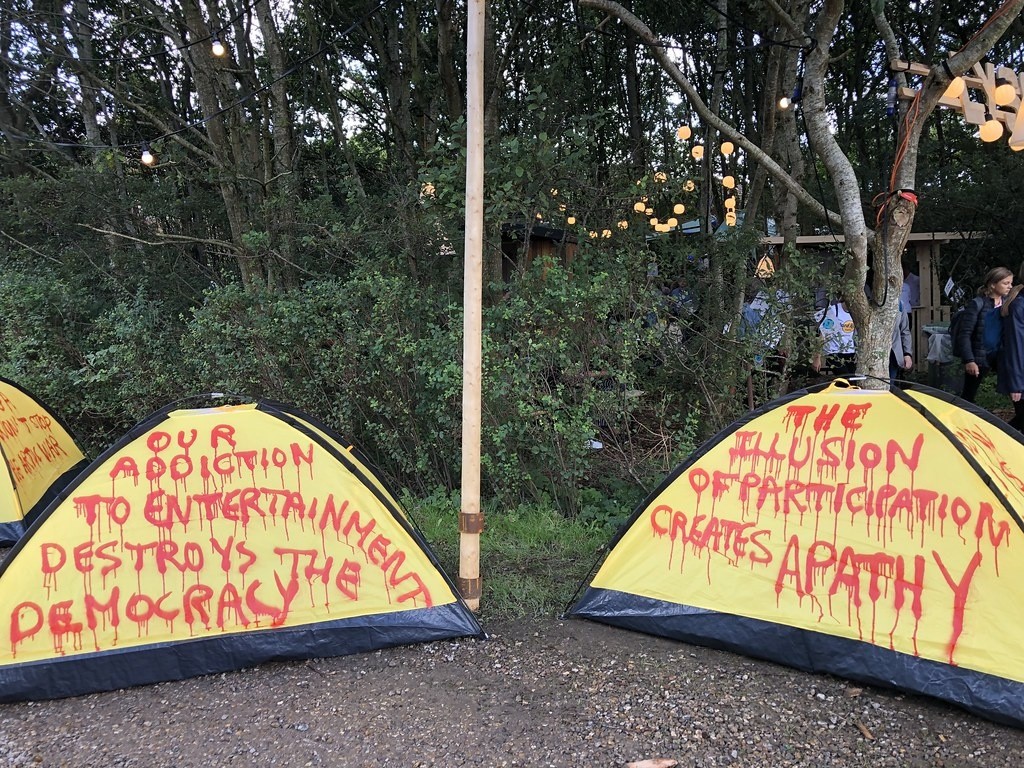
[0,393,486,706]
[563,374,1023,730]
[0,378,91,549]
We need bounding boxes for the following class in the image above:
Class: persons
[889,300,914,384]
[811,300,859,386]
[815,282,828,309]
[995,261,1024,432]
[900,283,913,330]
[948,267,1014,403]
[901,259,920,307]
[638,274,793,373]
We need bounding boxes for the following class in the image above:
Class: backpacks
[948,297,983,358]
